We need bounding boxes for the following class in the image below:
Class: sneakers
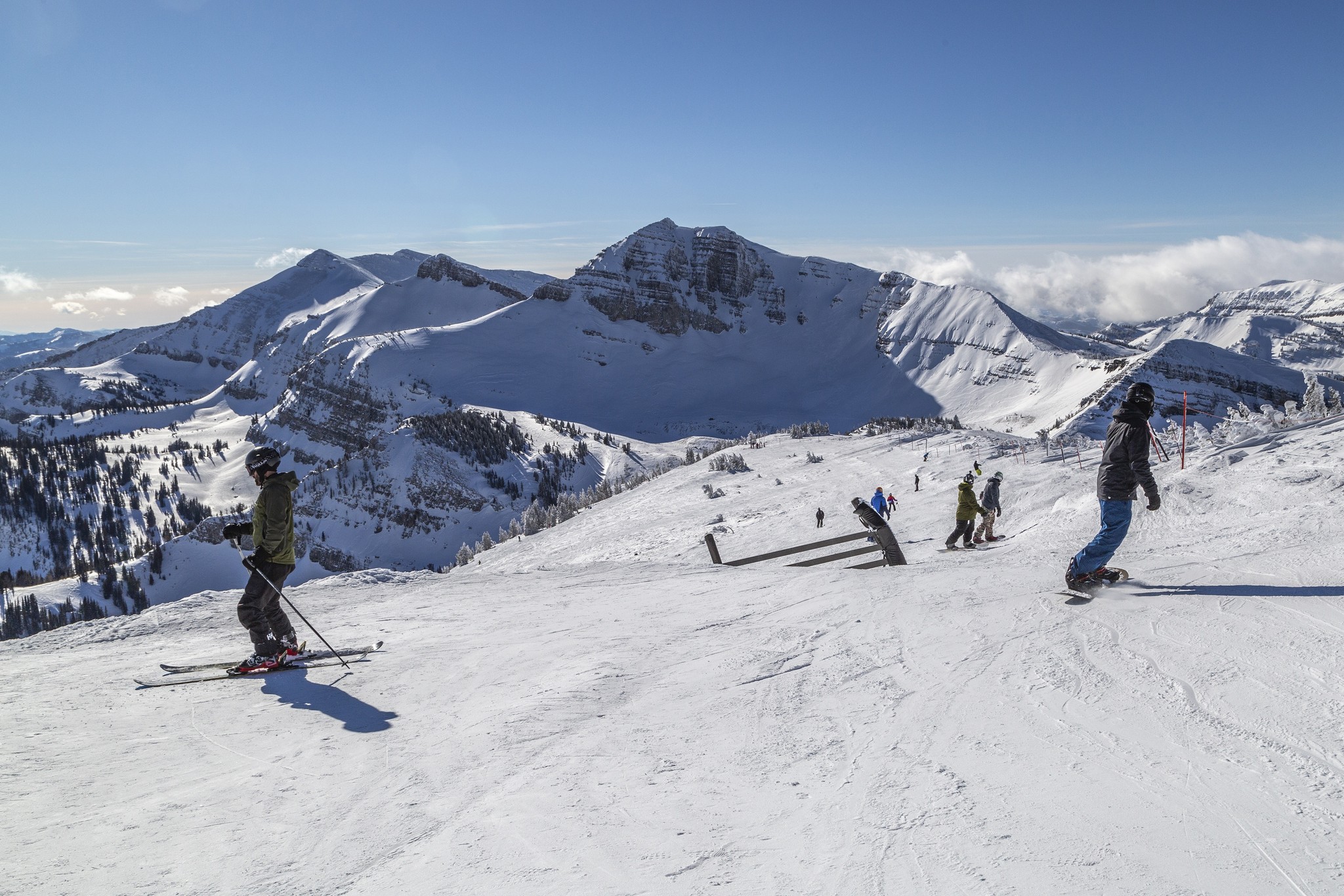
[1066,560,1103,592]
[963,541,976,548]
[867,537,871,541]
[985,534,998,542]
[946,542,959,550]
[1092,564,1120,581]
[271,627,297,659]
[973,536,984,544]
[227,653,300,674]
[870,537,875,542]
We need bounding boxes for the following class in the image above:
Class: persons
[973,471,1005,545]
[866,487,890,543]
[914,474,920,492]
[223,446,302,669]
[816,508,824,528]
[1065,382,1164,590]
[943,474,987,551]
[974,460,982,476]
[886,492,898,513]
[923,453,929,461]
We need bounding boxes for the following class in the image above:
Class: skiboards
[133,641,383,687]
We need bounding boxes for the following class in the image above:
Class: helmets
[818,507,820,510]
[915,474,917,476]
[993,471,1003,482]
[876,487,883,493]
[963,474,974,484]
[889,493,892,496]
[245,446,281,468]
[1127,382,1155,420]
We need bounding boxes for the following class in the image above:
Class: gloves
[223,522,251,540]
[242,545,271,571]
[981,512,987,517]
[996,510,1001,517]
[979,491,984,499]
[1146,494,1161,511]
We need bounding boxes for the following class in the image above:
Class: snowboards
[935,547,978,553]
[1054,567,1129,600]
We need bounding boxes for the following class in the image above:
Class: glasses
[245,465,254,476]
